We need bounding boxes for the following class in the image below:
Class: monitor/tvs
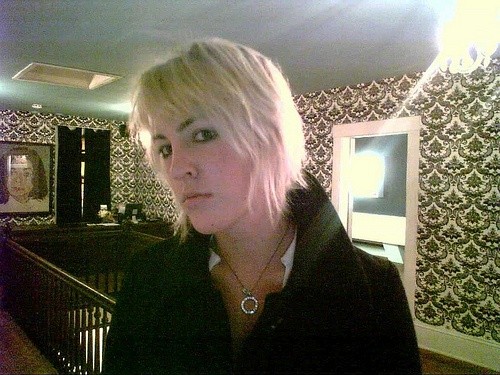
[125,203,143,216]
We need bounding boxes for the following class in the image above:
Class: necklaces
[212,216,291,315]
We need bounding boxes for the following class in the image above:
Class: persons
[102,36,423,375]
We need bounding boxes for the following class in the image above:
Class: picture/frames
[0,140,55,216]
